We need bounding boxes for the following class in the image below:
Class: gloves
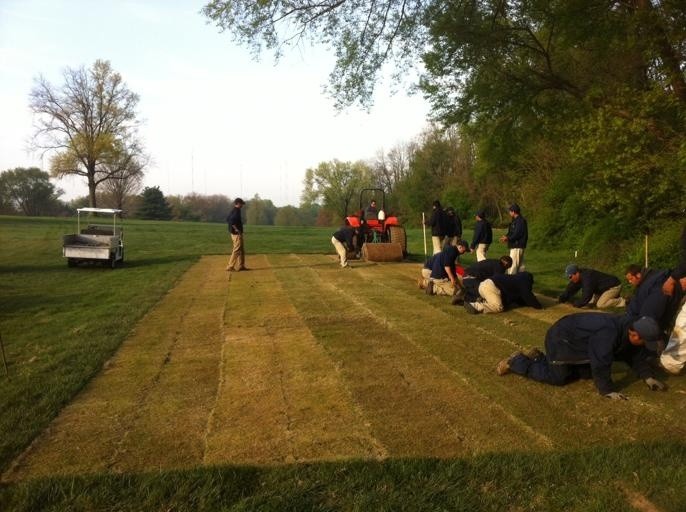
[646,377,665,391]
[605,392,628,401]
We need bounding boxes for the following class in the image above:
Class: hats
[459,240,471,252]
[567,264,579,274]
[633,315,659,351]
[234,198,245,204]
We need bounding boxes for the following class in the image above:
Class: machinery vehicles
[344,188,408,262]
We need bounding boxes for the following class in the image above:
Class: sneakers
[463,301,478,314]
[418,279,434,295]
[228,266,249,271]
[496,350,522,376]
[527,347,541,360]
[451,288,464,304]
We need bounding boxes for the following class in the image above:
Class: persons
[624,264,685,375]
[498,314,667,401]
[333,201,384,269]
[227,198,250,272]
[553,264,632,309]
[417,201,544,313]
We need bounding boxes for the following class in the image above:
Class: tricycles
[62,207,124,269]
